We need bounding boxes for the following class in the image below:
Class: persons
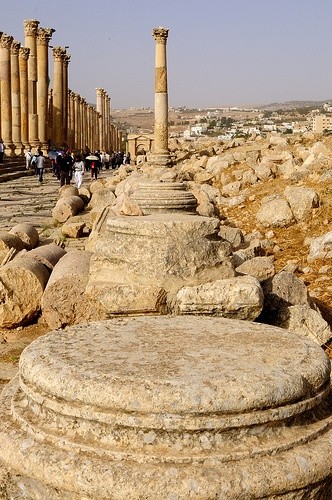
[0,139,133,189]
